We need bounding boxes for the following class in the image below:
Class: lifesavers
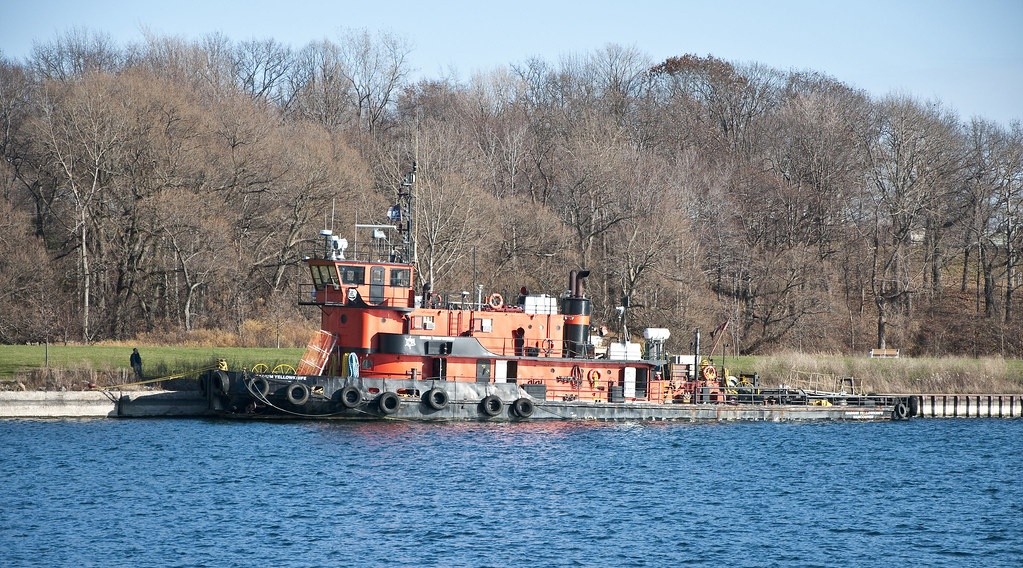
[702,366,716,380]
[588,369,602,382]
[896,403,906,418]
[428,387,449,410]
[341,385,363,408]
[483,395,504,416]
[542,338,555,353]
[428,294,442,306]
[247,376,270,400]
[212,371,230,395]
[287,382,310,406]
[515,398,534,418]
[489,293,503,309]
[379,391,401,415]
[197,374,208,397]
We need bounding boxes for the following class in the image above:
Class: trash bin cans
[611,386,623,403]
[523,384,546,401]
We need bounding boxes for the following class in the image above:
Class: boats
[197,133,907,426]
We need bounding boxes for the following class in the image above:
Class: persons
[130,348,142,378]
